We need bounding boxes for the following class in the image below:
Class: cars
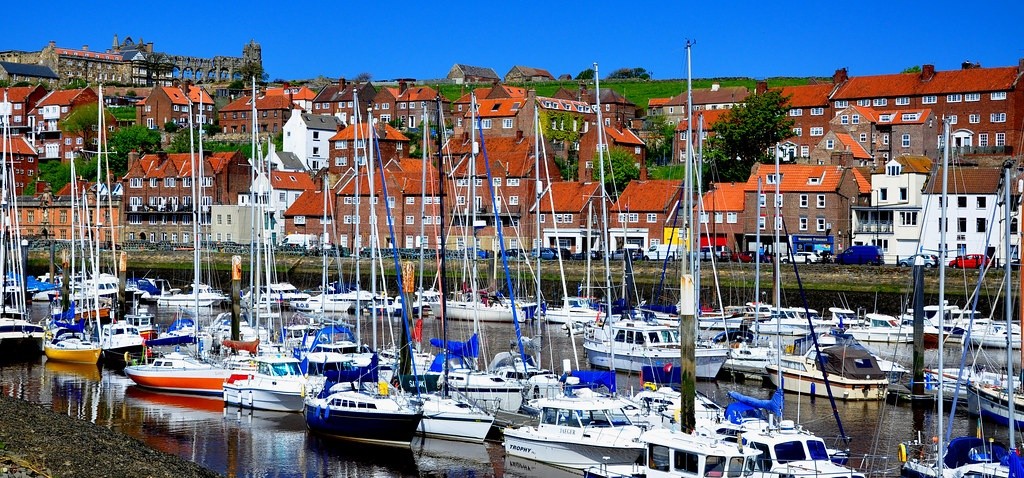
[611,248,638,260]
[571,248,598,260]
[501,248,524,263]
[781,252,823,265]
[463,247,487,260]
[952,255,991,269]
[733,251,758,262]
[900,253,938,269]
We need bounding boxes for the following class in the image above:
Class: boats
[966,379,1024,429]
[963,321,1021,350]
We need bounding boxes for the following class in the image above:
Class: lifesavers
[785,345,794,353]
[897,442,908,463]
[300,384,306,398]
[643,382,658,393]
[251,360,258,367]
[514,304,519,309]
[124,352,131,362]
[382,292,387,296]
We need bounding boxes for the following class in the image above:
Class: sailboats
[0,42,1024,478]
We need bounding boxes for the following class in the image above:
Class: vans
[688,245,728,262]
[532,247,558,260]
[644,245,681,261]
[836,246,884,266]
